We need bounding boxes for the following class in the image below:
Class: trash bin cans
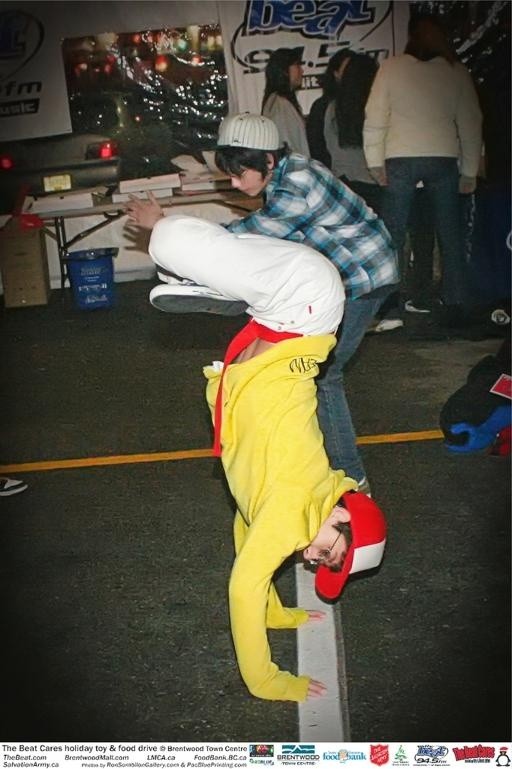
[62,246,117,311]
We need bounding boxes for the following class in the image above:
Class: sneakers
[149,282,249,316]
[0,475,27,496]
[366,317,404,334]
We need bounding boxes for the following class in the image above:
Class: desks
[21,190,263,302]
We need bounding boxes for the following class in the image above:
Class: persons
[322,54,381,216]
[307,48,355,170]
[363,15,483,334]
[124,112,402,504]
[148,215,387,704]
[404,137,495,314]
[260,46,310,160]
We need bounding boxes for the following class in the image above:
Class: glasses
[310,524,345,565]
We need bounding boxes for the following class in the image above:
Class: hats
[315,492,389,599]
[216,112,279,152]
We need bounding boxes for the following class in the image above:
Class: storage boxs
[0,226,50,308]
